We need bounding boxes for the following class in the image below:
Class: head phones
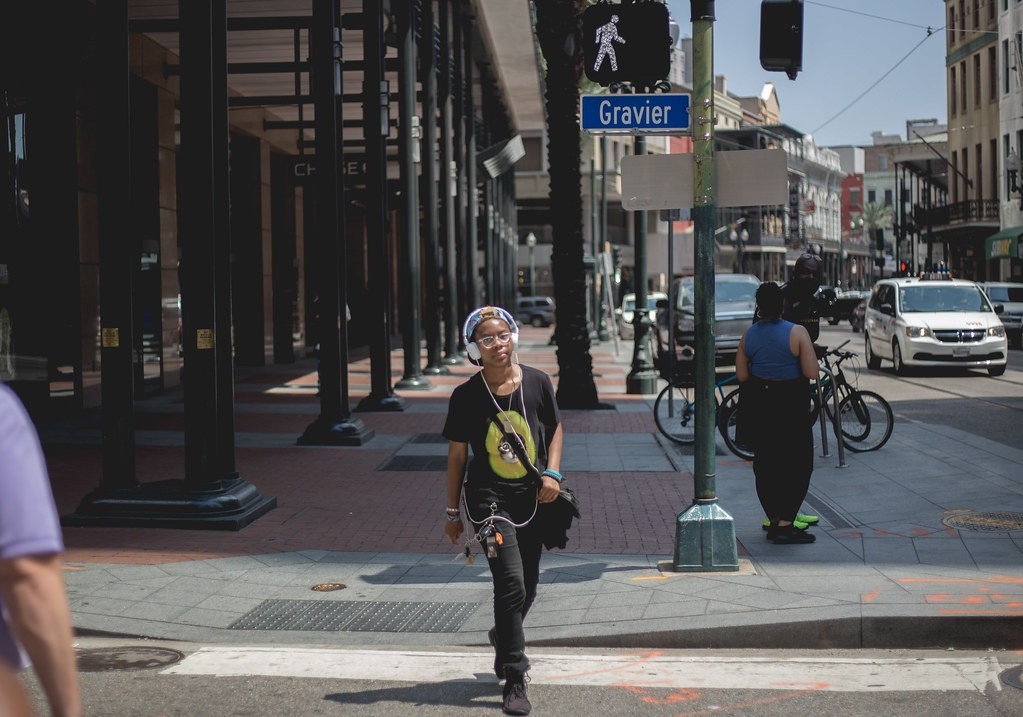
[463,306,520,360]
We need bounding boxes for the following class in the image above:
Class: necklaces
[491,373,515,417]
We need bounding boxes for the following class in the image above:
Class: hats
[466,307,513,366]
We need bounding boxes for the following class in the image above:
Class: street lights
[526,231,536,297]
[730,229,749,275]
[849,220,855,245]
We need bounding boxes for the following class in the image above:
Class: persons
[736,283,820,542]
[752,253,824,530]
[445,308,563,715]
[0,386,81,717]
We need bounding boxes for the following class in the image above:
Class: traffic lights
[580,1,671,84]
[613,246,621,268]
[900,257,908,276]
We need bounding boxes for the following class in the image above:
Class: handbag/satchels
[543,486,582,550]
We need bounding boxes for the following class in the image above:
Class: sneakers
[774,523,816,543]
[766,524,774,539]
[502,677,531,715]
[763,516,809,530]
[796,513,820,524]
[488,626,504,679]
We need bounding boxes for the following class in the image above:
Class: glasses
[477,332,513,349]
[801,253,822,261]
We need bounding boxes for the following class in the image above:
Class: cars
[865,271,1007,376]
[640,305,670,369]
[825,292,872,333]
[616,293,665,339]
[975,282,1022,349]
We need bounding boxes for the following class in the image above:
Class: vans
[655,275,760,383]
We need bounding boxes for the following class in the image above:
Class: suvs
[517,297,556,326]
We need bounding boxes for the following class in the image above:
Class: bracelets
[446,506,460,522]
[542,469,562,482]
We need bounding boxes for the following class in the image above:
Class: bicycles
[722,347,894,461]
[717,339,871,452]
[654,364,739,446]
